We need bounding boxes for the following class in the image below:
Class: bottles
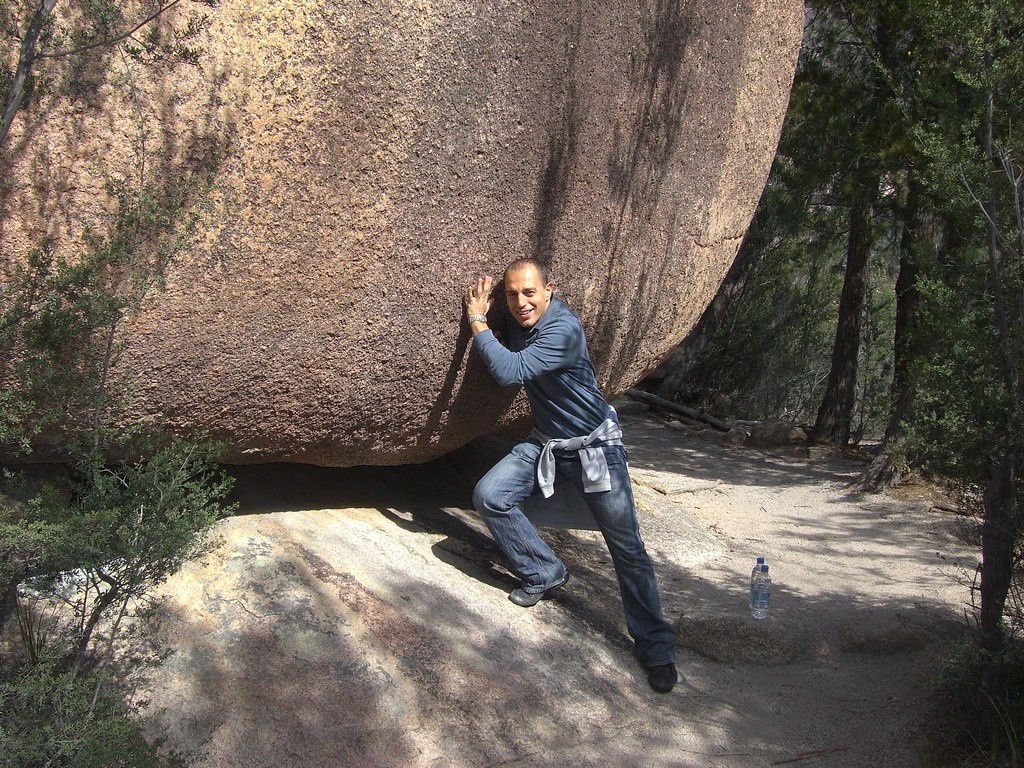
[753,565,772,619]
[750,558,764,608]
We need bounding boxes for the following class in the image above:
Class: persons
[466,258,678,694]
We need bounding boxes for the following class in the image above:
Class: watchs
[468,314,487,324]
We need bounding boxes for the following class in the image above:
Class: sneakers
[509,570,570,608]
[647,664,677,692]
